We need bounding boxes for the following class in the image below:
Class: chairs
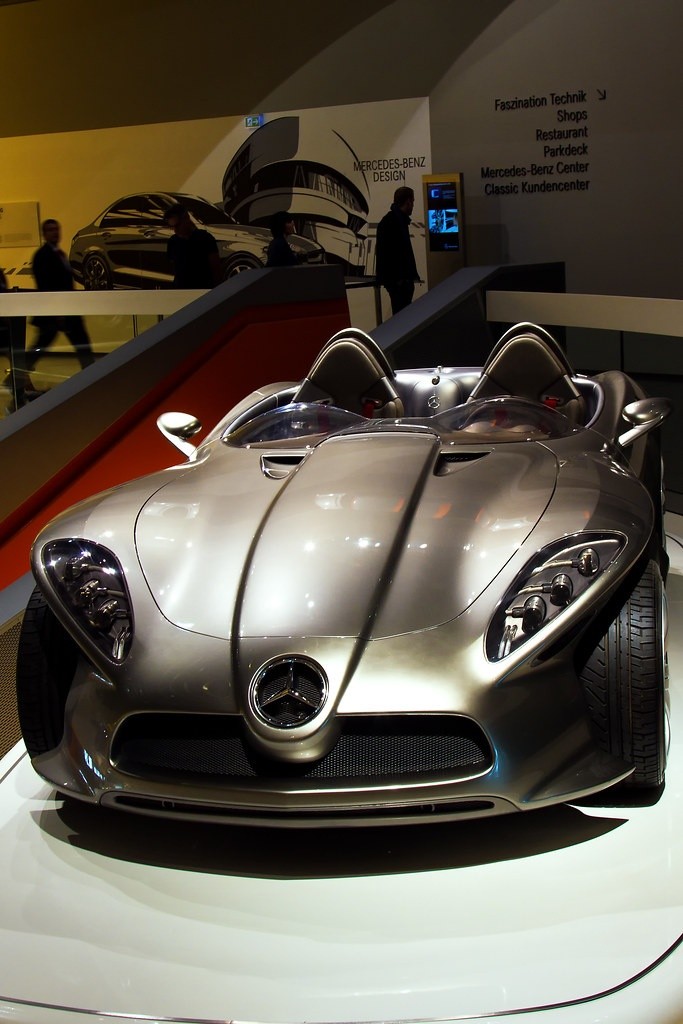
[456,320,588,442]
[281,326,405,436]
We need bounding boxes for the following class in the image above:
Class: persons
[0,267,53,415]
[164,203,221,290]
[3,219,96,388]
[265,209,325,270]
[376,187,420,318]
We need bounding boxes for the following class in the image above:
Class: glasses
[167,222,181,229]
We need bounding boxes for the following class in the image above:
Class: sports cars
[69,191,327,293]
[16,317,683,839]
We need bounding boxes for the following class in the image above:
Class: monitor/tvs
[427,182,459,252]
